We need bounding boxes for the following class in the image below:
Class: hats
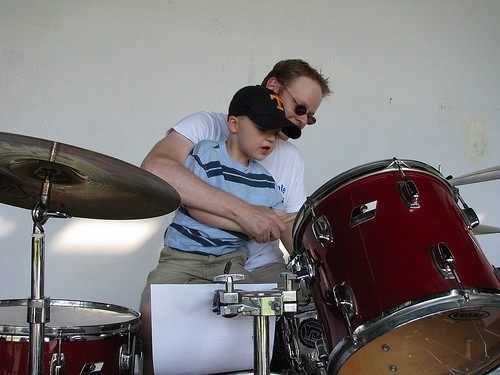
[228,85,302,139]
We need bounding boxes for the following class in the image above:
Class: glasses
[282,82,316,125]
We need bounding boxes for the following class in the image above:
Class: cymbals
[470,224,500,236]
[0,132,182,220]
[450,164,500,187]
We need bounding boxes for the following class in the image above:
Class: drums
[0,298,144,375]
[282,287,331,375]
[291,157,500,374]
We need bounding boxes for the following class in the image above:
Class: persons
[140,58,335,289]
[138,84,301,375]
